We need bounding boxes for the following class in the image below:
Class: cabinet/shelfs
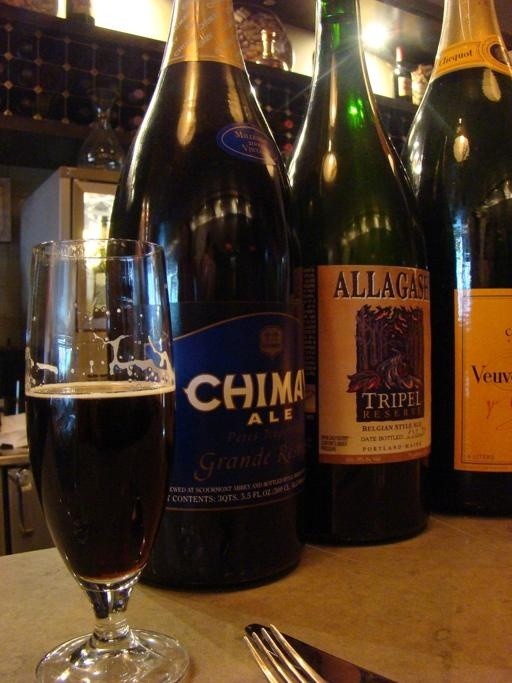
[1,4,420,170]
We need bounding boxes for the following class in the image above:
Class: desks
[0,411,511,683]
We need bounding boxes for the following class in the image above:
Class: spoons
[241,622,323,683]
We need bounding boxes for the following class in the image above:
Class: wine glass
[19,232,191,682]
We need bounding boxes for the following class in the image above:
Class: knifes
[243,622,399,683]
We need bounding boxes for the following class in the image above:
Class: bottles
[102,0,308,592]
[287,0,433,548]
[400,0,512,523]
